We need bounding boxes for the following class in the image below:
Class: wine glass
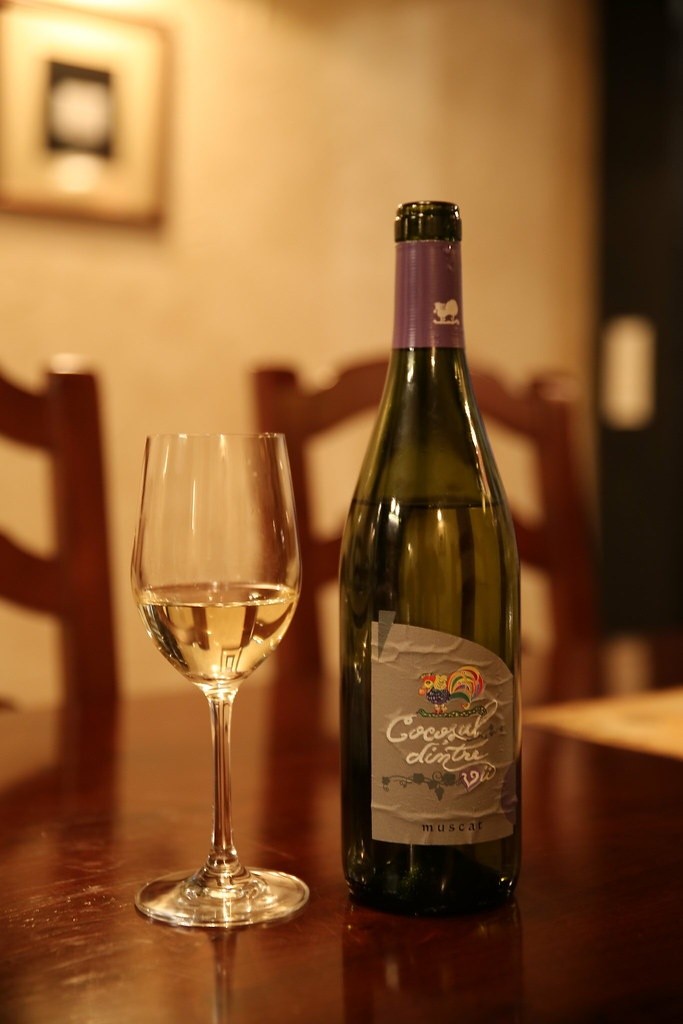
[129,429,311,929]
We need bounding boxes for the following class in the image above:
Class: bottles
[336,198,527,914]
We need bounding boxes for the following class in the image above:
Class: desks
[0,664,683,1023]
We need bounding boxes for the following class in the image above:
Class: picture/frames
[1,5,175,228]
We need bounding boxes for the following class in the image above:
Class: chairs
[0,352,128,723]
[246,350,610,632]
